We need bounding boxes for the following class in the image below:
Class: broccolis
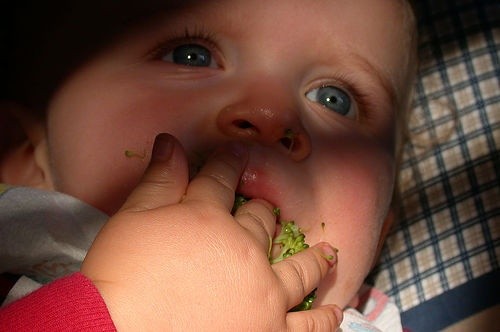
[230,195,319,310]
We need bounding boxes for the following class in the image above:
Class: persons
[1,1,418,332]
